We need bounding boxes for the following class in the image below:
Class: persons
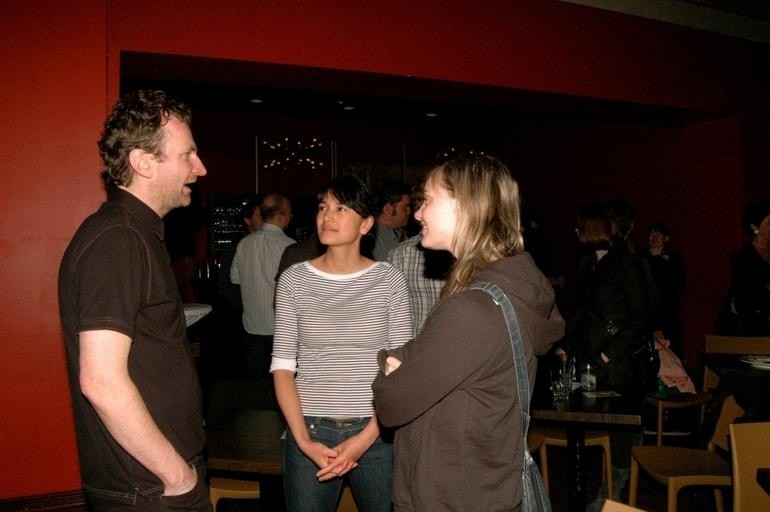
[267,176,415,508]
[361,185,456,331]
[722,194,770,336]
[368,148,573,511]
[545,200,684,404]
[227,188,327,414]
[54,86,220,509]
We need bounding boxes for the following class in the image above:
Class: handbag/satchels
[521,454,552,512]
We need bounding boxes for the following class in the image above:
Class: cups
[581,363,598,391]
[551,367,570,402]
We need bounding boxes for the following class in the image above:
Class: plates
[739,354,770,371]
